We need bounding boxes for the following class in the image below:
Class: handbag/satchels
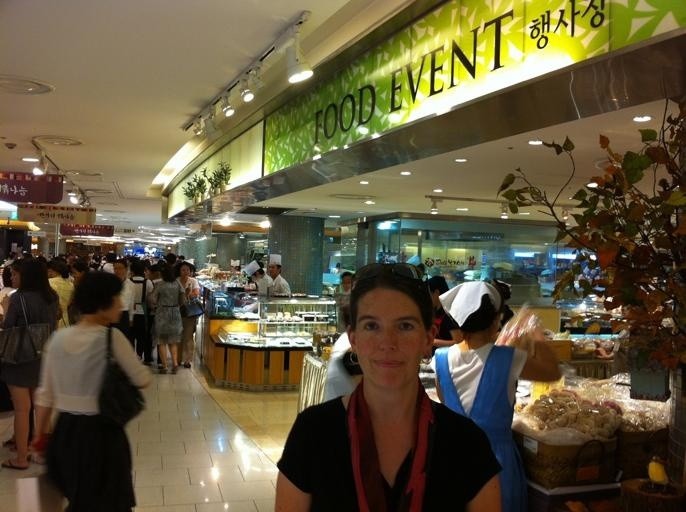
[0,292,51,365]
[98,324,148,430]
[180,292,206,319]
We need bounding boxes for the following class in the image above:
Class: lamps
[424,195,587,220]
[21,139,91,208]
[182,10,314,137]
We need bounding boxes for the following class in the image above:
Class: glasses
[349,262,432,305]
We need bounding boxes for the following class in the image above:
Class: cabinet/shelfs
[195,271,342,391]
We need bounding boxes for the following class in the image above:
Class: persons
[28,271,152,512]
[276,263,562,511]
[1,253,201,469]
[243,253,291,298]
[593,304,637,375]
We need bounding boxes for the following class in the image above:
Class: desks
[298,351,686,512]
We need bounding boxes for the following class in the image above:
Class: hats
[243,259,262,278]
[231,258,240,266]
[268,253,283,266]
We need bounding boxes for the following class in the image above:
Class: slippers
[1,434,48,470]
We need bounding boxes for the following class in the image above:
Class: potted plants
[492,68,686,512]
[181,159,232,205]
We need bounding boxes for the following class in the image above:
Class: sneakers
[138,354,192,374]
